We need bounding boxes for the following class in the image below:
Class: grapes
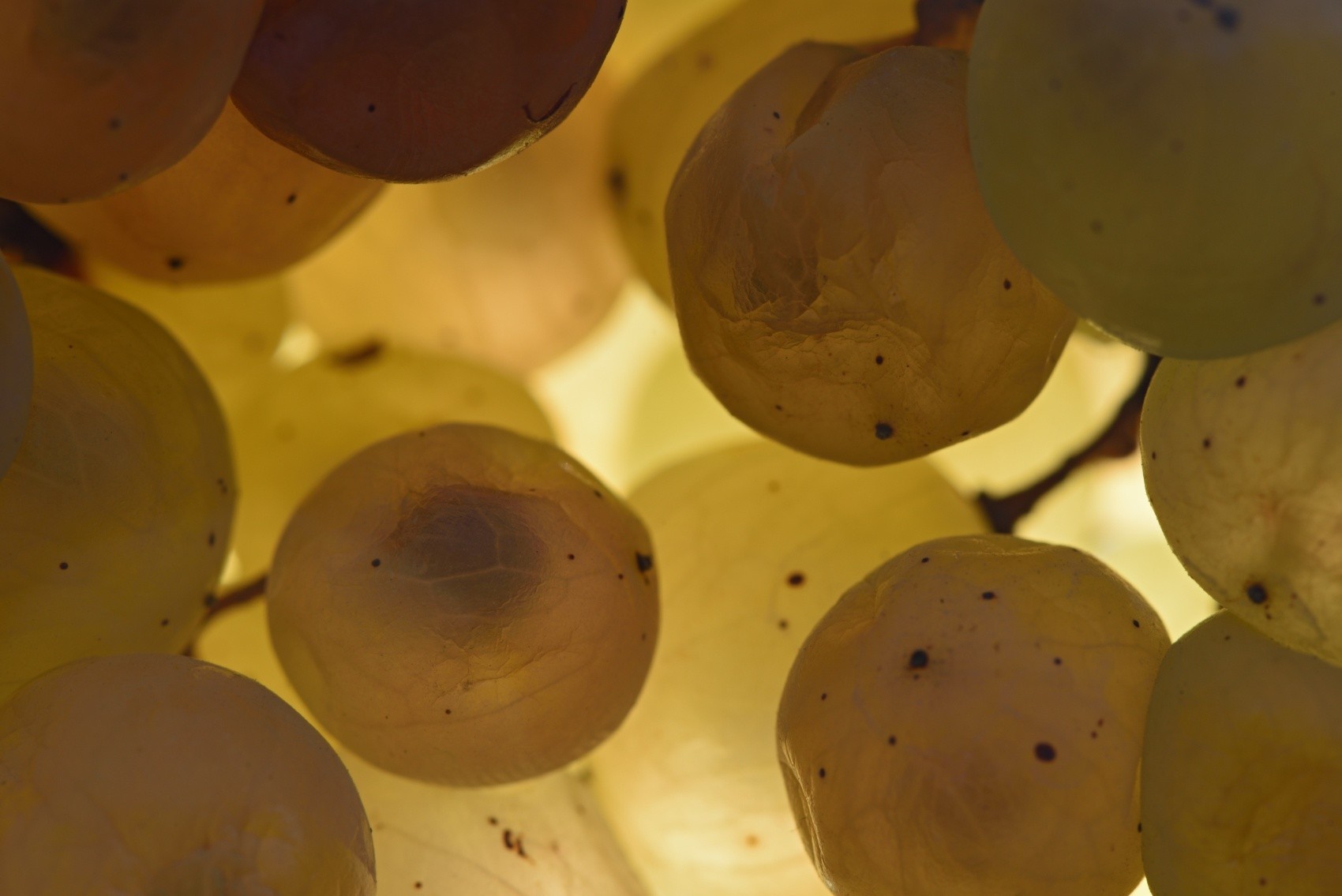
[0,0,1342,896]
